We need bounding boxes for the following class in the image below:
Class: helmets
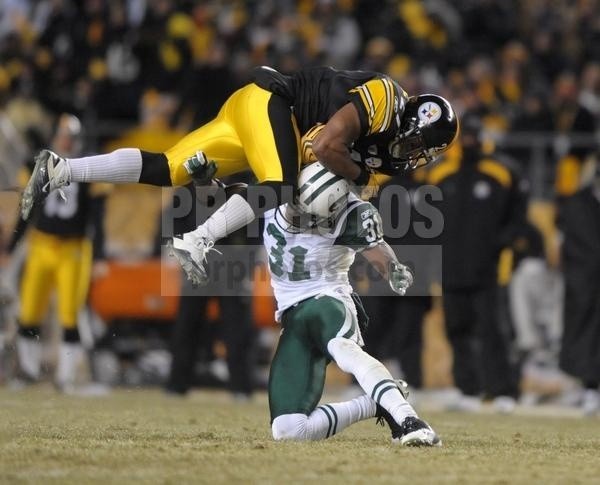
[274,160,349,235]
[390,94,457,171]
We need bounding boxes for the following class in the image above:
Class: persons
[1,2,600,418]
[181,146,444,449]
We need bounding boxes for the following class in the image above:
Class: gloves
[183,151,219,185]
[388,260,415,297]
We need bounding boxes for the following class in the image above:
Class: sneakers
[165,232,210,288]
[376,379,442,447]
[21,148,69,221]
